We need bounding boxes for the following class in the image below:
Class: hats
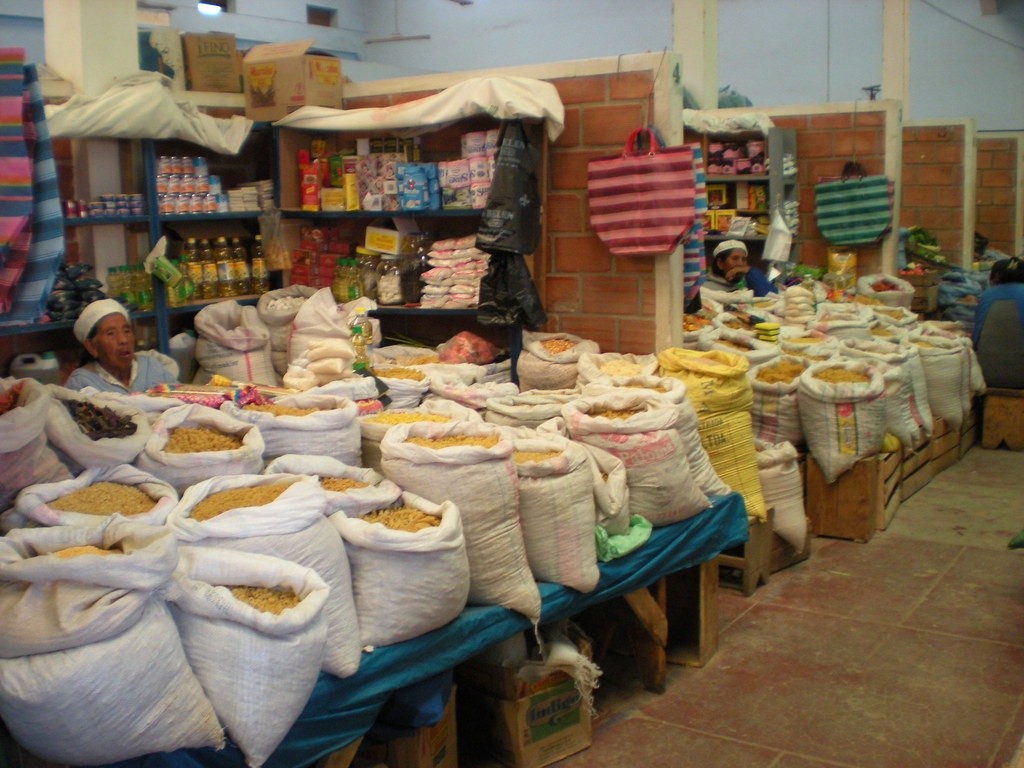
[73,297,129,344]
[713,239,748,257]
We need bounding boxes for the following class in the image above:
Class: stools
[715,502,777,596]
[980,387,1024,453]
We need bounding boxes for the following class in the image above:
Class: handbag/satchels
[813,163,894,248]
[587,126,709,309]
[1,43,62,334]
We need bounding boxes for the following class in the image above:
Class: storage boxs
[901,270,939,311]
[180,31,242,93]
[378,682,458,767]
[493,661,593,768]
[242,40,342,122]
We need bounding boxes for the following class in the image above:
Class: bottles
[333,230,436,303]
[108,236,271,314]
[348,308,374,376]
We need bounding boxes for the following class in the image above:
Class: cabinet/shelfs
[1,96,548,342]
[704,122,800,281]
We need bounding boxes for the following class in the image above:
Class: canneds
[62,194,149,219]
[154,155,229,214]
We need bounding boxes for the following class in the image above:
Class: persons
[684,239,778,314]
[970,256,1024,390]
[64,299,179,395]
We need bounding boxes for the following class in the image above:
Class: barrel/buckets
[169,329,197,385]
[10,351,60,387]
